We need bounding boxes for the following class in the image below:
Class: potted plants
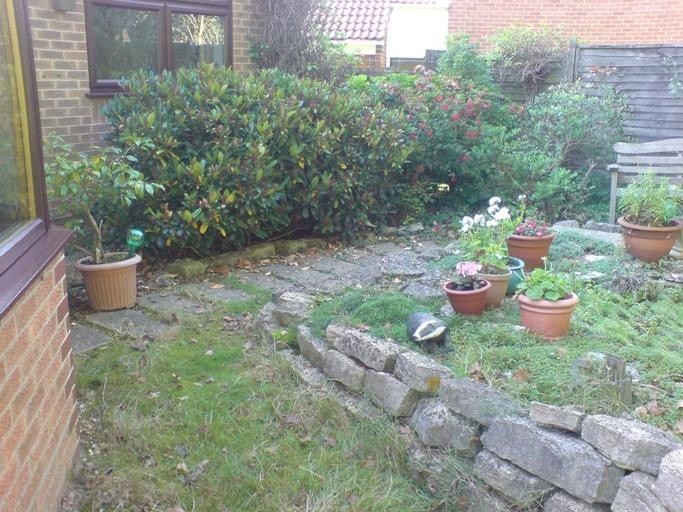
[39,129,167,312]
[615,158,683,265]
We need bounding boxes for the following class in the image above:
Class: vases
[442,231,557,317]
[517,288,580,344]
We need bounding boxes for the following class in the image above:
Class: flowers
[511,254,575,303]
[448,192,549,290]
